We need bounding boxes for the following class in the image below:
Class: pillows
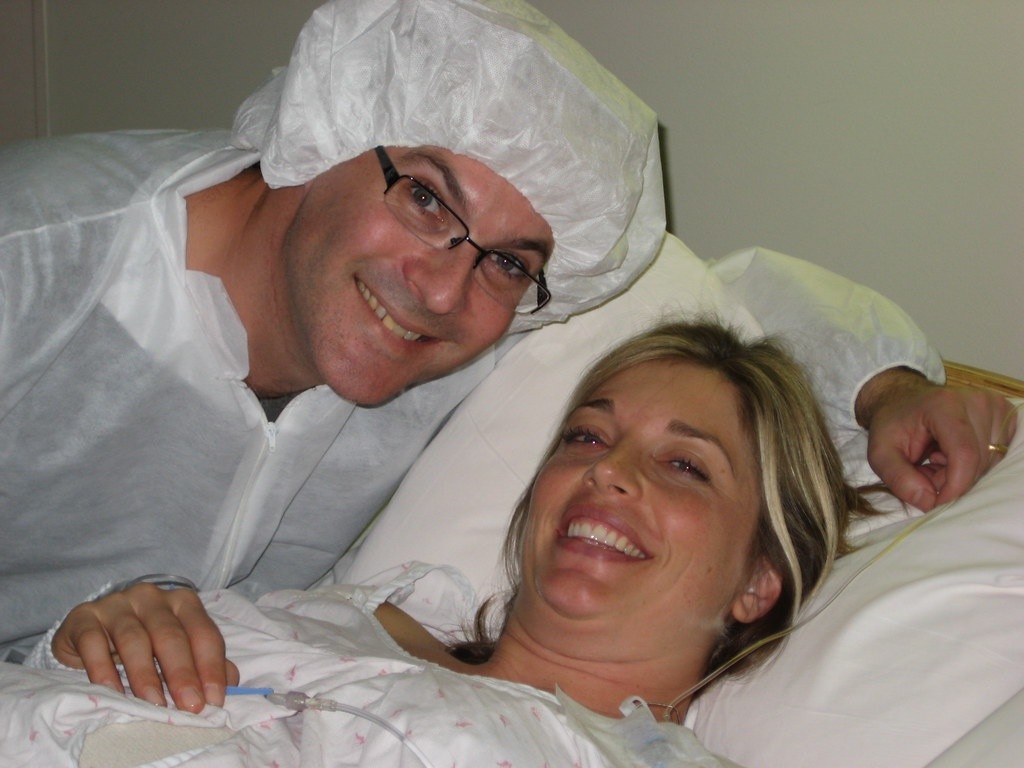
[320,229,1024,768]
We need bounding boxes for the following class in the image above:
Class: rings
[987,444,1009,454]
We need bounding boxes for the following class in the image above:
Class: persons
[0,298,913,768]
[1,0,1019,665]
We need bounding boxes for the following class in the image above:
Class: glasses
[375,145,552,315]
